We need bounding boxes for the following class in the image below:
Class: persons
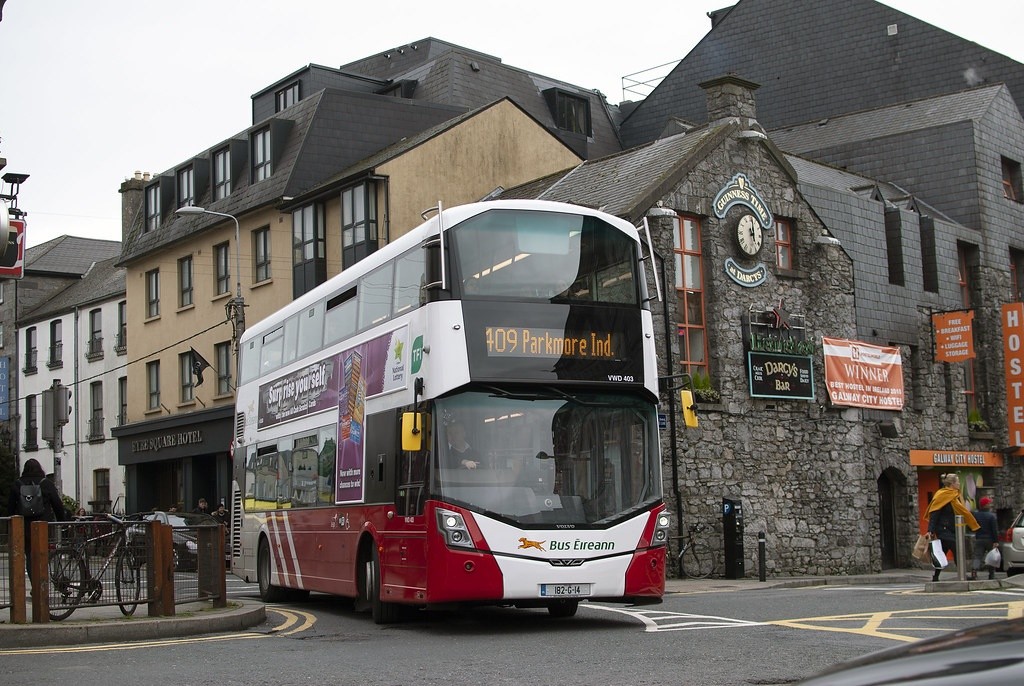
[445,418,487,470]
[93,507,110,557]
[191,497,209,515]
[169,503,178,513]
[72,506,89,559]
[970,496,1000,580]
[212,502,231,536]
[924,472,977,582]
[10,457,71,598]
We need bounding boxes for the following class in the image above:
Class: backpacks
[21,485,45,517]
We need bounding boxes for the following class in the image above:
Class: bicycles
[666,521,716,580]
[48,510,142,620]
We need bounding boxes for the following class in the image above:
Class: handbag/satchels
[914,537,948,570]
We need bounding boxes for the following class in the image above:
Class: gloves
[461,460,477,469]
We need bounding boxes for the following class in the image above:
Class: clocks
[735,212,764,256]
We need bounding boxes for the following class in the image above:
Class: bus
[229,198,699,623]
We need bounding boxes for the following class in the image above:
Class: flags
[188,346,210,388]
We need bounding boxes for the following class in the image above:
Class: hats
[979,497,992,507]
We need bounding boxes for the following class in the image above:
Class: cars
[126,512,231,572]
[1001,509,1024,578]
